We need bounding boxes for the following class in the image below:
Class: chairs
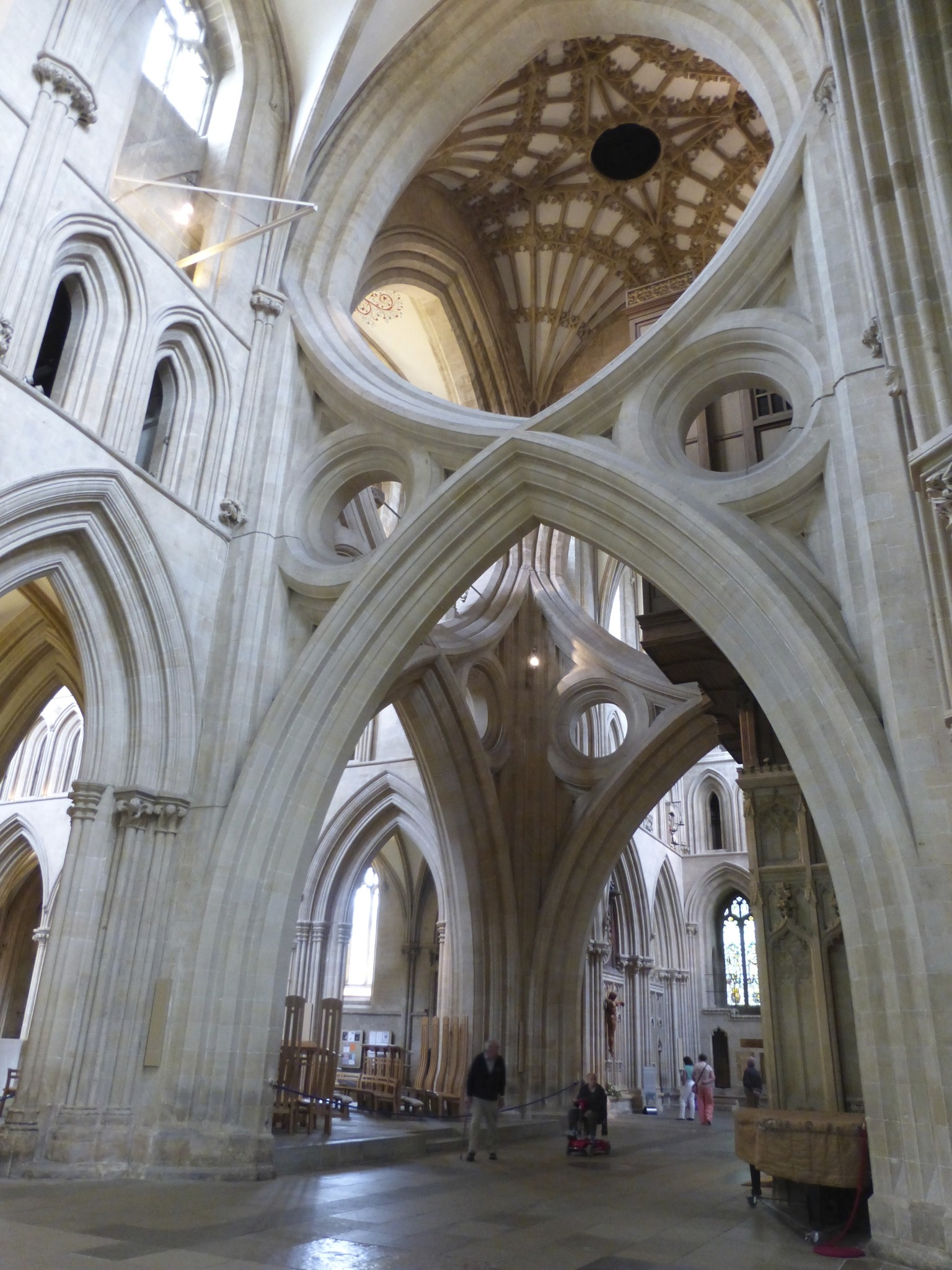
[270,995,474,1135]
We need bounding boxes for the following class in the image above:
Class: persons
[692,1053,716,1125]
[464,1039,506,1162]
[604,991,625,1060]
[676,1056,696,1121]
[567,1073,607,1143]
[743,1056,763,1109]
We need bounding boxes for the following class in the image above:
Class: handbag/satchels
[691,1083,698,1094]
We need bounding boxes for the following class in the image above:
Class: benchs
[0,1067,21,1118]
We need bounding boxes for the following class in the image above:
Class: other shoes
[675,1118,685,1120]
[686,1118,694,1120]
[588,1137,596,1144]
[489,1153,496,1160]
[466,1152,477,1162]
[706,1120,712,1125]
